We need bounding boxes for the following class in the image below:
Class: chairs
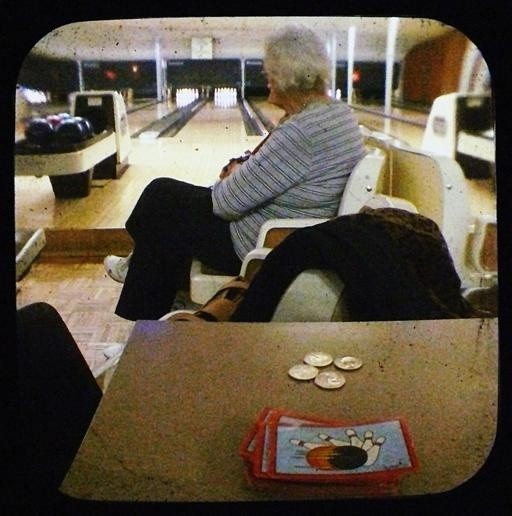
[7,297,105,511]
[183,147,419,311]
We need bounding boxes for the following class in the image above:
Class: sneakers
[104,252,131,283]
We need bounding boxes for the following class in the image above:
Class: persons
[102,23,369,362]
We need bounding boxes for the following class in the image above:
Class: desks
[58,299,505,504]
